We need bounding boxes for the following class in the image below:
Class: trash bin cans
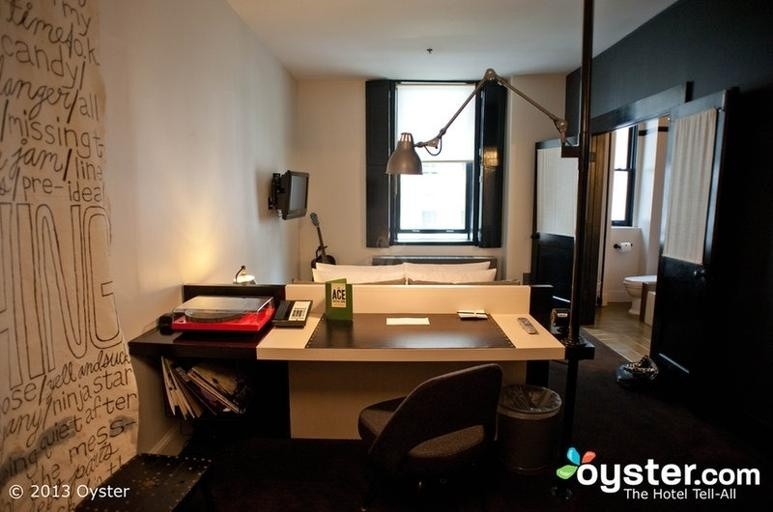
[496,383,562,471]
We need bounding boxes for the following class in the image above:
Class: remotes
[518,317,537,333]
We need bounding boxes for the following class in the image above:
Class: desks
[125,280,567,440]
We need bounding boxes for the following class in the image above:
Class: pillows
[310,260,497,284]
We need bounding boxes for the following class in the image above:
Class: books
[159,355,249,421]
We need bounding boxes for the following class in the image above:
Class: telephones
[272,299,313,328]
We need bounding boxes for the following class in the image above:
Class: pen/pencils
[457,312,487,314]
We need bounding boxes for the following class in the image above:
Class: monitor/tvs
[269,169,310,220]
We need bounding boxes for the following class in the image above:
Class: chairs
[358,363,515,512]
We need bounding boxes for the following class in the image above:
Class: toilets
[622,274,658,316]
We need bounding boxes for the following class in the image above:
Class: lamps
[383,68,566,175]
